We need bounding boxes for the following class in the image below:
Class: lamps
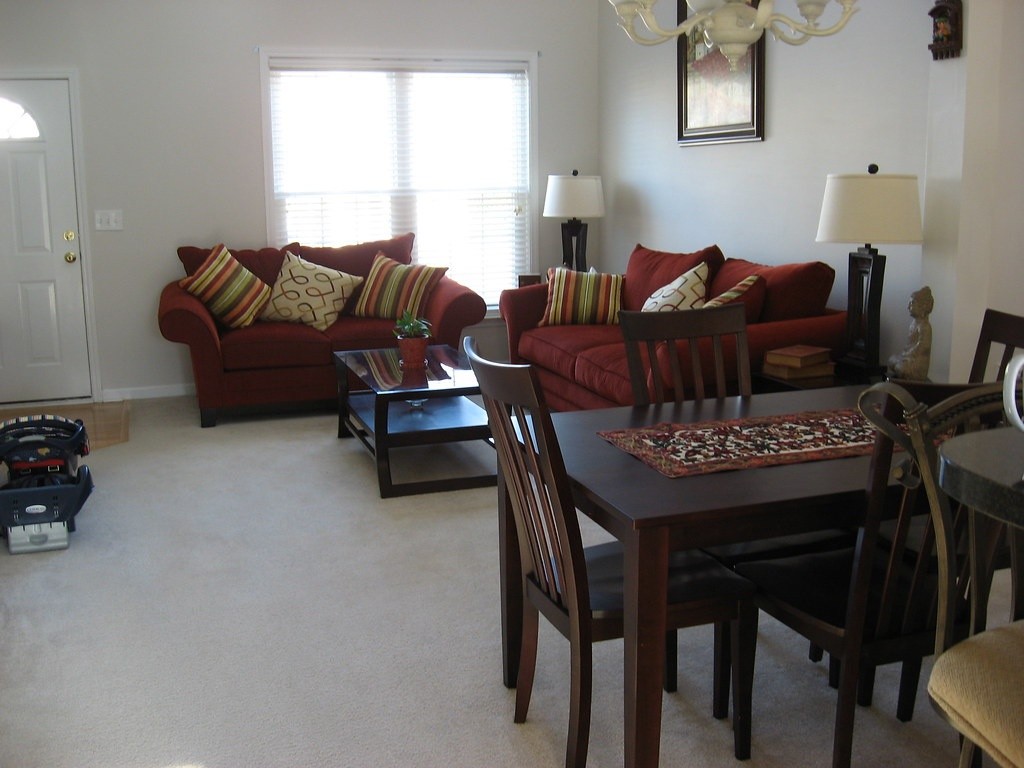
[609,0,859,73]
[543,170,606,270]
[814,165,925,383]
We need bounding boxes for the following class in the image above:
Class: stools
[926,619,1024,768]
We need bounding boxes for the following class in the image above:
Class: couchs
[498,260,852,415]
[155,264,486,427]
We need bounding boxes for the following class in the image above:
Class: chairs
[734,378,1024,767]
[614,304,852,717]
[873,307,1024,581]
[462,335,756,767]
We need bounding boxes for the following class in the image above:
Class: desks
[938,424,1024,638]
[485,382,953,767]
[722,352,888,394]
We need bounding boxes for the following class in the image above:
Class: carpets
[0,397,130,453]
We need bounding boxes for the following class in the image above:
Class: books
[763,361,835,380]
[765,343,832,368]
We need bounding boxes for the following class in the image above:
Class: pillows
[349,250,450,320]
[707,257,835,321]
[537,266,623,326]
[174,240,300,286]
[620,243,726,310]
[299,232,414,313]
[701,272,767,324]
[257,250,366,332]
[178,242,273,328]
[641,261,709,310]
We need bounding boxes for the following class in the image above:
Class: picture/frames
[674,0,765,148]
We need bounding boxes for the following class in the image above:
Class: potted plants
[390,309,433,361]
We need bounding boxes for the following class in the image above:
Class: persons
[888,287,933,380]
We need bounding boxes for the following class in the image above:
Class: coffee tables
[332,343,500,500]
